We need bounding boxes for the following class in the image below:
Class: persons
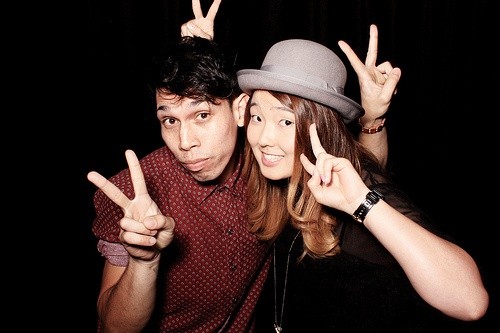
[176,0,490,333]
[86,22,401,333]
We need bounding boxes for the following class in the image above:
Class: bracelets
[349,185,384,223]
[355,112,388,134]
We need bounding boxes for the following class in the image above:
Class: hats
[236,39,365,122]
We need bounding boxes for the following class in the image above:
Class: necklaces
[271,225,303,333]
